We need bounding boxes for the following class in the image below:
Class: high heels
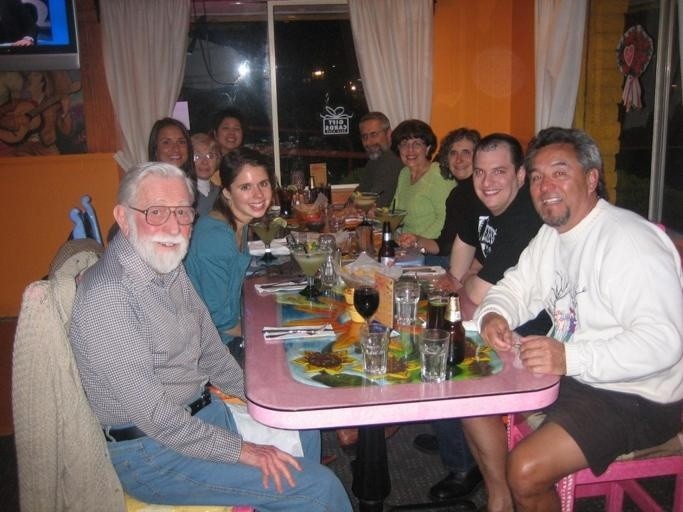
[337,425,401,453]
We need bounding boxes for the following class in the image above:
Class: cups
[354,271,451,383]
[321,251,342,290]
[278,189,293,215]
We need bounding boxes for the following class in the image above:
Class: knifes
[262,329,335,333]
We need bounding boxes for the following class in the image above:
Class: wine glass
[375,207,407,248]
[290,245,331,298]
[352,192,380,226]
[249,219,283,262]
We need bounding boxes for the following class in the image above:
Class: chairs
[505,221,683,512]
[21,194,259,512]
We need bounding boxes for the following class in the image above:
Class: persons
[210,110,244,188]
[394,126,484,272]
[69,162,355,512]
[460,126,683,512]
[189,132,222,215]
[147,117,199,208]
[0,0,38,47]
[371,121,457,239]
[412,132,552,500]
[340,112,405,210]
[183,146,401,466]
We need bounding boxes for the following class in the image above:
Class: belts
[101,387,212,443]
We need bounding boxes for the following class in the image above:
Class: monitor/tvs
[0,0,81,72]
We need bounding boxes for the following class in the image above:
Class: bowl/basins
[307,222,325,230]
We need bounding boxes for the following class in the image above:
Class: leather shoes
[427,472,484,502]
[412,432,440,455]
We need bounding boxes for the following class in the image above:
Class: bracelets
[415,240,418,248]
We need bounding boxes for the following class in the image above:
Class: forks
[266,325,327,340]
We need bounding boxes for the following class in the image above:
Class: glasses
[127,205,198,226]
[192,153,216,163]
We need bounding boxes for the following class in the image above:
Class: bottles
[379,222,395,265]
[309,176,330,203]
[443,293,466,364]
